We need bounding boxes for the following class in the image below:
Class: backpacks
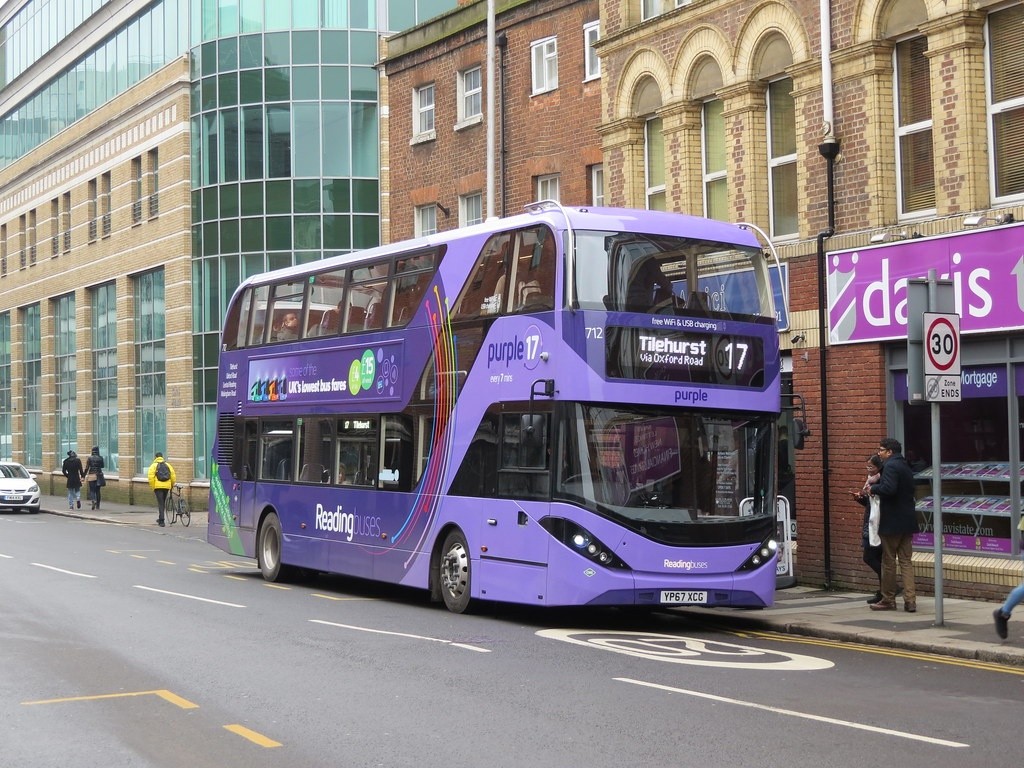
[156,460,170,482]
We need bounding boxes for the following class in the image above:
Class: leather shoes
[865,591,882,603]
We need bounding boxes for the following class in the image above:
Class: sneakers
[993,606,1011,641]
[903,598,916,612]
[869,595,897,610]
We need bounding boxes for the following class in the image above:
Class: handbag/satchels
[85,473,98,481]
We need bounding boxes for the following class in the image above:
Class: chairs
[250,272,562,486]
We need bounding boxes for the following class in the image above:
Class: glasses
[865,465,876,469]
[878,448,889,452]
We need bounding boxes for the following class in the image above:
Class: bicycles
[165,483,190,528]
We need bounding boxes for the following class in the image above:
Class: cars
[0,461,42,514]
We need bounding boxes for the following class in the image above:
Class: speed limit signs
[921,310,962,402]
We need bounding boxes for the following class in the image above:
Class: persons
[62,450,85,509]
[851,454,904,604]
[993,514,1024,638]
[81,447,106,510]
[148,452,176,527]
[867,438,918,613]
[276,312,300,339]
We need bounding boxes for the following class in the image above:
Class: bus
[206,197,813,623]
